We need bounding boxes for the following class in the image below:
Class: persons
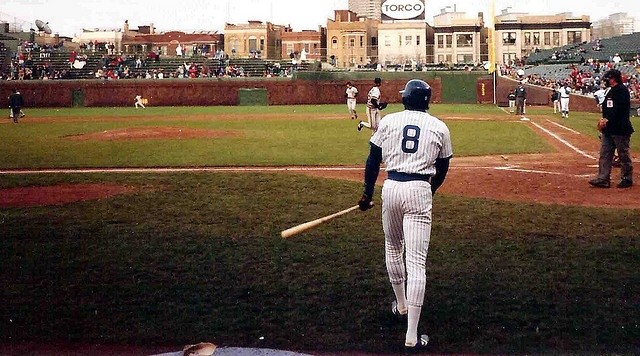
[627,54,640,99]
[254,51,259,58]
[577,48,586,53]
[552,49,571,60]
[34,40,89,80]
[249,51,254,59]
[551,90,561,114]
[134,94,146,109]
[170,44,245,79]
[528,75,571,88]
[514,82,527,115]
[359,79,453,350]
[262,49,303,77]
[10,88,25,118]
[501,57,526,81]
[358,78,388,136]
[10,90,23,123]
[95,54,165,80]
[594,79,611,113]
[79,39,116,54]
[600,53,621,68]
[593,40,602,53]
[600,62,627,80]
[507,89,516,116]
[559,83,571,118]
[571,59,600,95]
[588,68,635,188]
[345,82,359,119]
[6,41,34,79]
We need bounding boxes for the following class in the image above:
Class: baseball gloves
[597,118,608,132]
[378,102,388,110]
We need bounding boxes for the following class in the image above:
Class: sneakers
[588,178,610,187]
[403,334,429,355]
[617,179,632,188]
[392,300,408,324]
[358,121,363,130]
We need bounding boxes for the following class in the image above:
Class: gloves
[358,194,372,211]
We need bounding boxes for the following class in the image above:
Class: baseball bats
[281,201,375,239]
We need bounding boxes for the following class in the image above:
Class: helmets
[399,79,431,110]
[604,69,621,87]
